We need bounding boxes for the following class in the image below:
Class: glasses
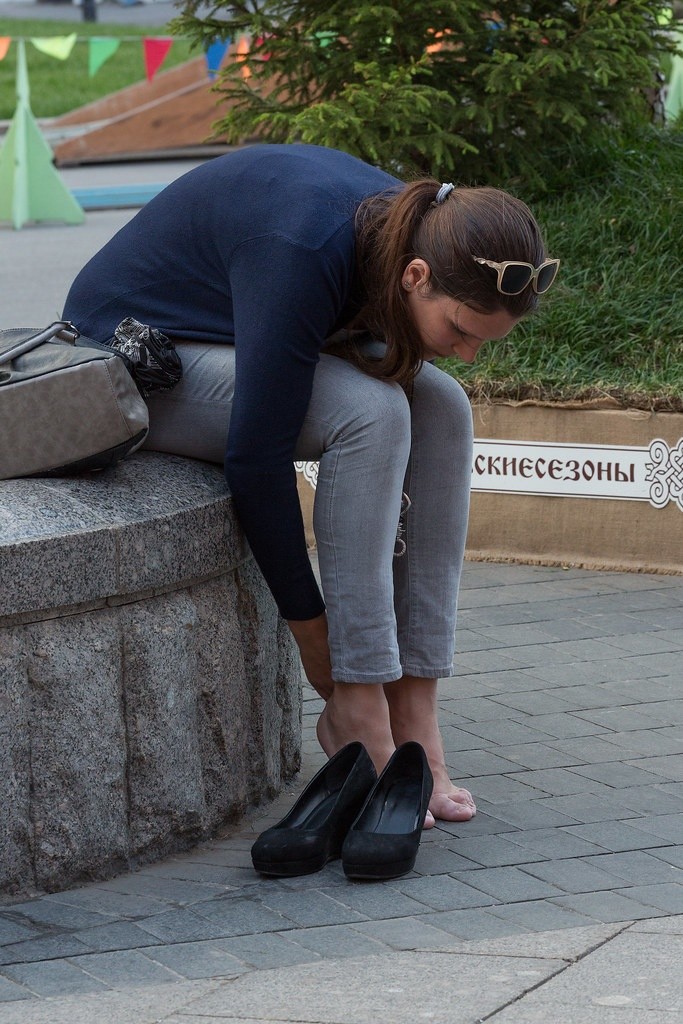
[471,255,561,295]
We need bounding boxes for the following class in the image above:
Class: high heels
[251,741,377,876]
[342,741,434,879]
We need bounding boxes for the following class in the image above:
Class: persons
[58,142,561,830]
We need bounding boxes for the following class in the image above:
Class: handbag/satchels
[0,321,149,480]
